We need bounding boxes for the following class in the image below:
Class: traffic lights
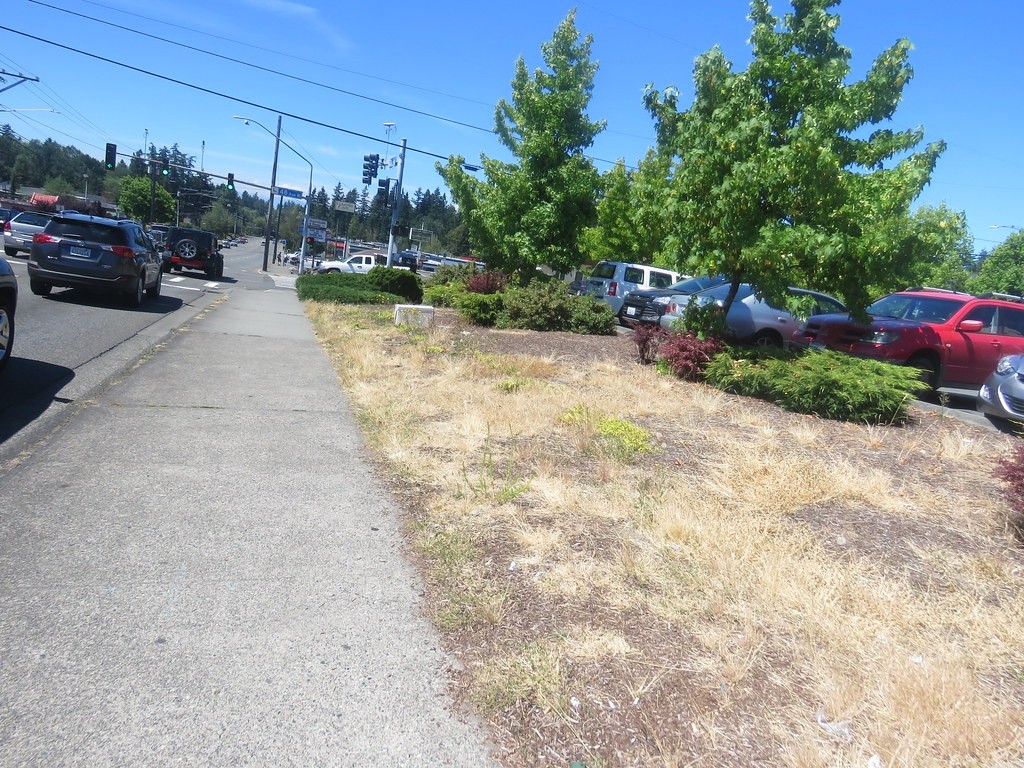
[361,154,372,185]
[162,158,170,177]
[367,152,379,178]
[228,173,235,191]
[104,143,117,171]
[377,178,391,205]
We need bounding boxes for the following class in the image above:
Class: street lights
[233,115,314,276]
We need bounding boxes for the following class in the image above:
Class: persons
[277,251,288,266]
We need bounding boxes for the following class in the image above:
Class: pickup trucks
[317,254,411,275]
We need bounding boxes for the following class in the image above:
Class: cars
[659,283,855,350]
[0,255,19,373]
[977,351,1024,428]
[217,236,247,250]
[0,207,20,231]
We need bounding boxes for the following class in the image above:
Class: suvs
[619,273,750,329]
[790,286,1024,402]
[3,212,62,256]
[27,210,166,309]
[585,260,705,315]
[160,226,224,280]
[393,254,417,273]
